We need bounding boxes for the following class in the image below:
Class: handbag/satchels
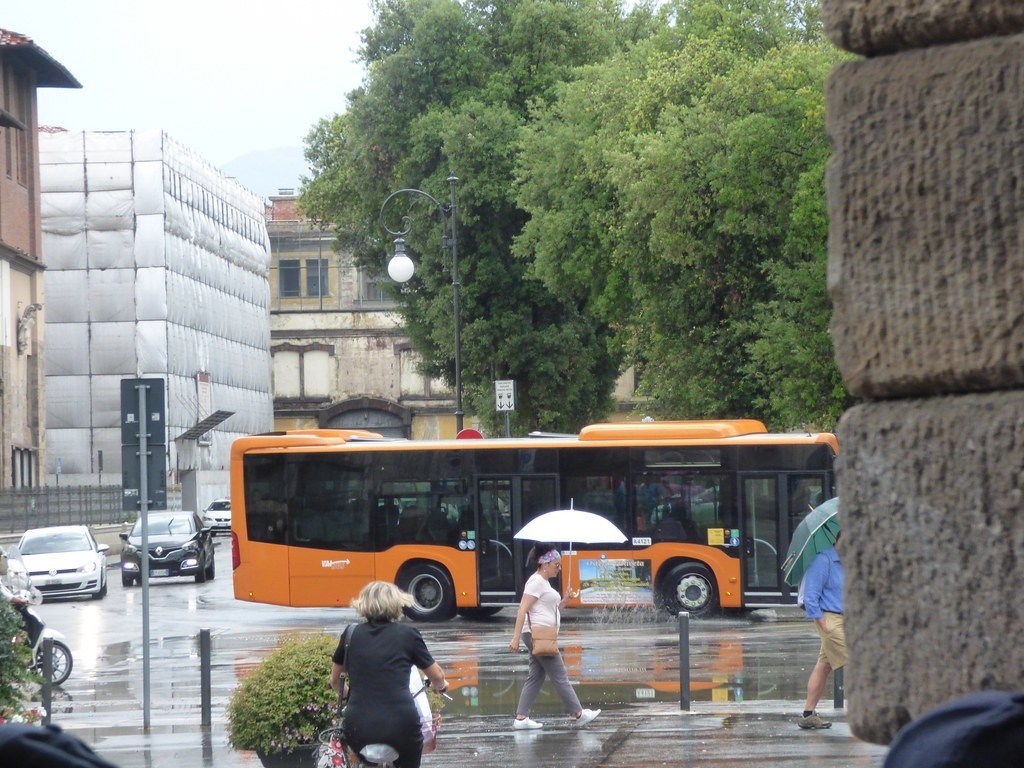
[409,664,432,733]
[316,717,362,768]
[532,626,559,656]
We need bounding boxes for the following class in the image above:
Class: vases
[255,741,321,768]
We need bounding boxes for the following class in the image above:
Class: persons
[510,540,601,730]
[331,582,449,768]
[799,529,847,728]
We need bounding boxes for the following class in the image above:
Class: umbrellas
[513,498,628,598]
[785,496,841,586]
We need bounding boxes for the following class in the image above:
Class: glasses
[549,562,559,568]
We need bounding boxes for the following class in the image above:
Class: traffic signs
[496,381,515,413]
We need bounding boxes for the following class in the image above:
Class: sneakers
[798,712,832,729]
[514,716,543,729]
[576,708,601,727]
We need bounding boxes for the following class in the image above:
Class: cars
[201,499,231,535]
[17,525,109,603]
[651,484,724,527]
[117,511,215,587]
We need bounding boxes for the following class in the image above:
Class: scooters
[12,545,74,687]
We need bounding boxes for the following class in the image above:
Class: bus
[226,426,840,624]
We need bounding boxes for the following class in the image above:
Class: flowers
[227,633,446,754]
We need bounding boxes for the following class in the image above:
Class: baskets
[420,713,440,754]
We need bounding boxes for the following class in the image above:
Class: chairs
[283,494,451,546]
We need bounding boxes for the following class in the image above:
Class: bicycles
[362,678,454,768]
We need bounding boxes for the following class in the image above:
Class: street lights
[378,173,468,440]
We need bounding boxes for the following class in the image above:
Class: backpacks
[797,553,830,610]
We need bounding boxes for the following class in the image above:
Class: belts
[821,609,841,614]
